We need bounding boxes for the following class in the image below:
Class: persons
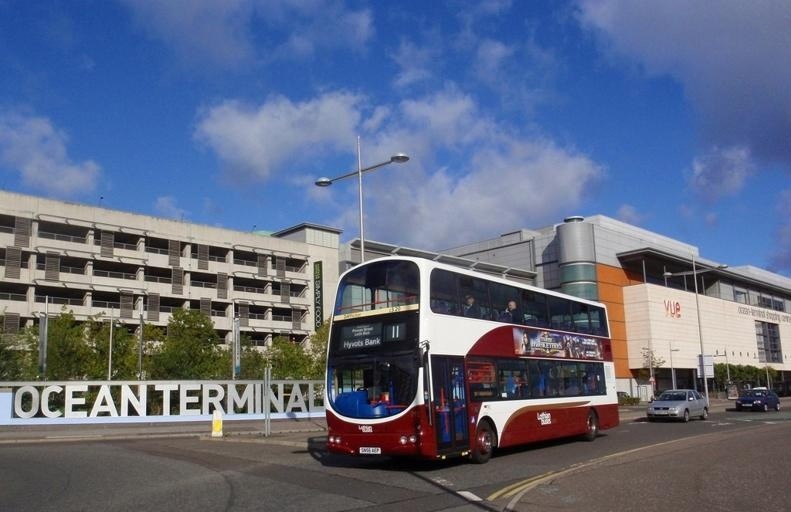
[377,291,606,397]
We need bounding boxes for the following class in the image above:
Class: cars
[736,385,782,413]
[646,389,711,422]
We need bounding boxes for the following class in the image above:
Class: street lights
[315,130,411,311]
[641,343,791,397]
[664,252,730,409]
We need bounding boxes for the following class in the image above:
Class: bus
[323,253,623,464]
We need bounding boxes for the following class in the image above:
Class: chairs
[334,375,604,443]
[432,301,607,339]
